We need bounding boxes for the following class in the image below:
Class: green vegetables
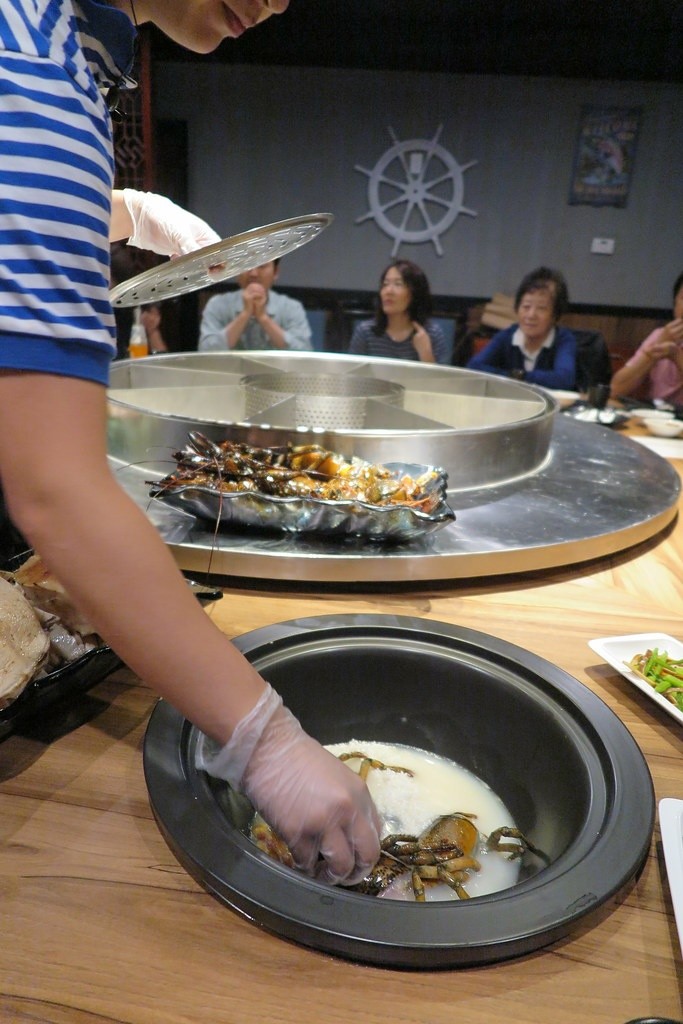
[631,647,683,712]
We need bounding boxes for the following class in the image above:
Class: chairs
[336,307,467,365]
[461,325,613,395]
[308,306,337,349]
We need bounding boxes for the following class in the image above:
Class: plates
[630,409,675,421]
[150,452,457,545]
[0,547,223,743]
[658,796,683,958]
[588,633,683,721]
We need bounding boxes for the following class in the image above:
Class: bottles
[130,324,148,358]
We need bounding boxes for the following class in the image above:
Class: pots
[96,334,555,490]
[148,616,655,966]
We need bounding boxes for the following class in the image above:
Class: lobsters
[113,428,432,573]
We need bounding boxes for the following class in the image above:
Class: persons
[349,261,450,367]
[466,267,577,391]
[0,0,383,887]
[610,272,683,412]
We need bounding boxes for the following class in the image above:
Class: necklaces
[106,0,138,116]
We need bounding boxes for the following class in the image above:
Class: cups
[587,385,611,410]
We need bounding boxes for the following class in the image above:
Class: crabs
[253,750,536,899]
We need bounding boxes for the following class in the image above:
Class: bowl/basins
[645,418,683,437]
[552,389,580,409]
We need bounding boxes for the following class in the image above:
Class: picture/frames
[568,105,643,209]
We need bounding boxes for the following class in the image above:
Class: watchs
[511,368,527,381]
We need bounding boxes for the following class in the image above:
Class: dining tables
[1,347,682,1024]
